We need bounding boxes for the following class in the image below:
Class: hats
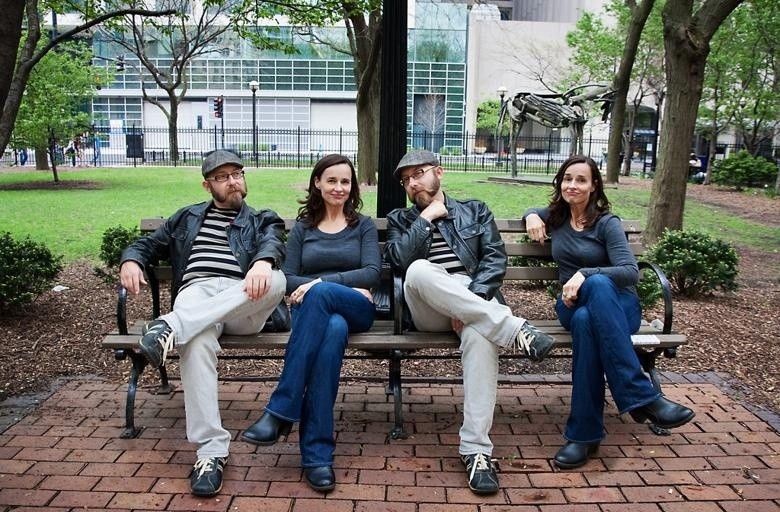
[393,145,438,182]
[198,147,243,176]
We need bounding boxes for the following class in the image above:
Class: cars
[75,136,90,149]
[2,145,15,160]
[203,144,242,158]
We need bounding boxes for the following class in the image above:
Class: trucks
[604,128,661,163]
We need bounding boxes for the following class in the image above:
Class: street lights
[737,97,747,150]
[650,89,665,171]
[248,80,259,162]
[494,87,509,167]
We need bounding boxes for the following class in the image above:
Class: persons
[118,149,287,498]
[239,154,382,493]
[521,154,696,471]
[384,150,558,496]
[596,146,608,173]
[690,150,700,171]
[13,130,103,167]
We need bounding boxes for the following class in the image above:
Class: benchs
[97,215,406,445]
[392,212,690,442]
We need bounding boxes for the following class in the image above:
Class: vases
[514,147,525,154]
[475,146,487,153]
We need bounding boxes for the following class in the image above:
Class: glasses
[399,167,434,187]
[207,171,243,183]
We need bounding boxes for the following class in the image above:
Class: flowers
[473,139,489,147]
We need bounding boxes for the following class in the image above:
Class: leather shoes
[241,410,293,446]
[552,436,603,467]
[626,394,696,430]
[301,461,337,492]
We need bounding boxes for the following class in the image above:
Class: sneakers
[514,317,556,364]
[458,448,501,495]
[189,449,230,497]
[137,317,178,369]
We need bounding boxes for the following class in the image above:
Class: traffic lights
[116,55,124,72]
[213,99,218,111]
[94,73,101,91]
[215,97,223,118]
